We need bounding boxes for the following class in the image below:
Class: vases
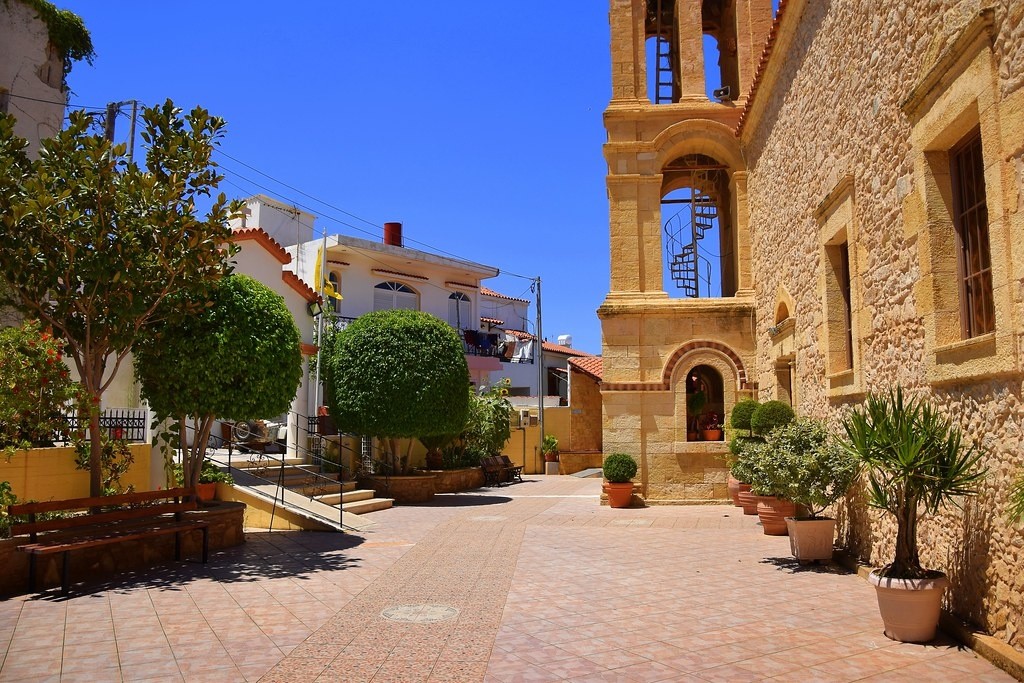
[702,429,722,440]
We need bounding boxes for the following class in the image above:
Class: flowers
[701,409,720,429]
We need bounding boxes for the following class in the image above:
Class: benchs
[8,488,212,596]
[479,455,525,487]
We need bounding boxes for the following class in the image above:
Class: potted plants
[839,385,990,643]
[171,457,234,501]
[602,450,638,509]
[756,397,802,537]
[542,435,558,462]
[726,398,760,510]
[687,391,703,440]
[739,403,791,514]
[752,417,856,562]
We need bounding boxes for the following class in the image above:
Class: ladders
[656,0,675,104]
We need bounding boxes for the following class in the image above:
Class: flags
[315,240,344,302]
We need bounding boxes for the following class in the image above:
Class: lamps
[307,302,323,317]
